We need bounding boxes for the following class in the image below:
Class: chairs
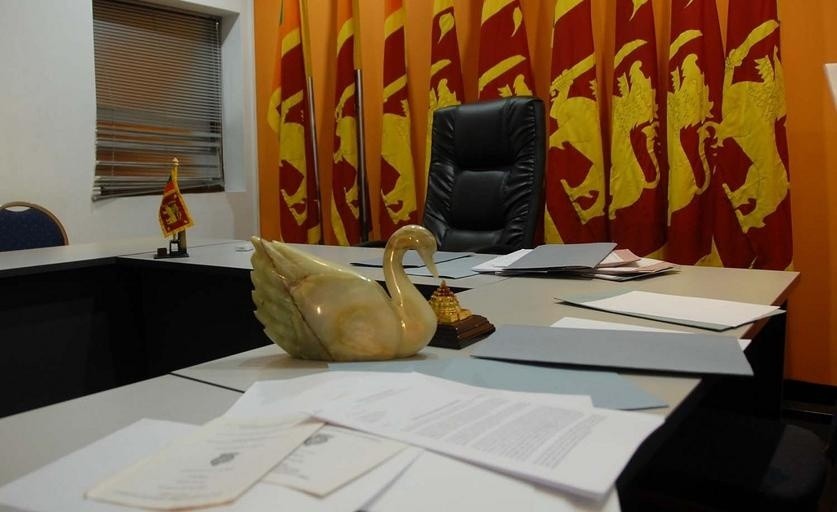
[419,95,546,256]
[0,201,69,252]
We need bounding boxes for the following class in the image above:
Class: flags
[476,0,538,108]
[158,171,194,238]
[667,2,725,269]
[718,0,787,265]
[613,1,659,263]
[328,0,361,245]
[545,1,609,243]
[277,0,321,246]
[423,0,466,209]
[379,0,416,239]
